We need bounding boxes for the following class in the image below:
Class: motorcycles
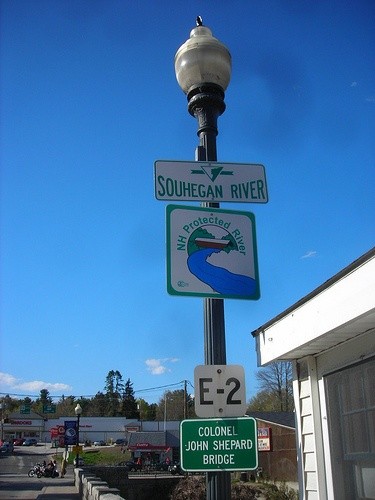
[47,461,59,478]
[28,460,50,478]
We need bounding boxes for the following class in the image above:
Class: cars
[14,438,26,445]
[23,439,37,446]
[94,440,104,445]
[0,441,13,456]
[116,439,124,443]
[5,438,15,441]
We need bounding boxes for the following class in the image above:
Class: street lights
[172,12,233,496]
[75,404,82,467]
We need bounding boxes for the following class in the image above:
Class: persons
[36,460,57,473]
